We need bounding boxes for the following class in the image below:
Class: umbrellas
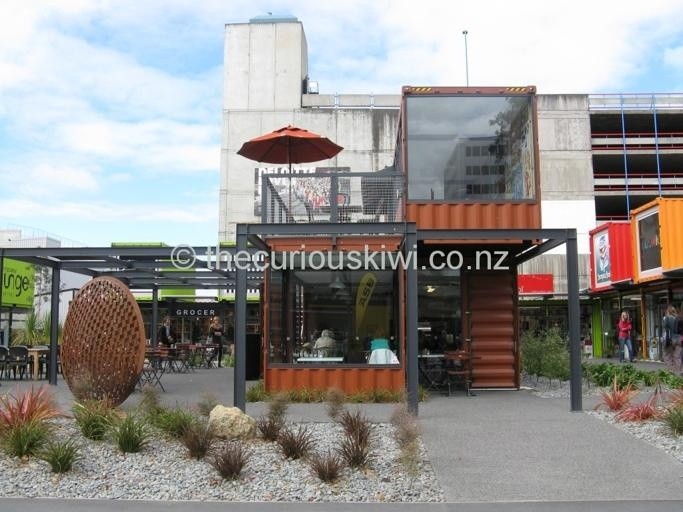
[237,121,344,210]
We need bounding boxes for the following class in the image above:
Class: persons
[367,326,391,354]
[617,312,638,362]
[312,328,337,357]
[661,304,681,371]
[206,315,226,367]
[157,315,176,370]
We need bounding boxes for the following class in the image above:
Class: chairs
[302,196,384,224]
[137,339,219,393]
[0,344,63,380]
[418,338,473,397]
